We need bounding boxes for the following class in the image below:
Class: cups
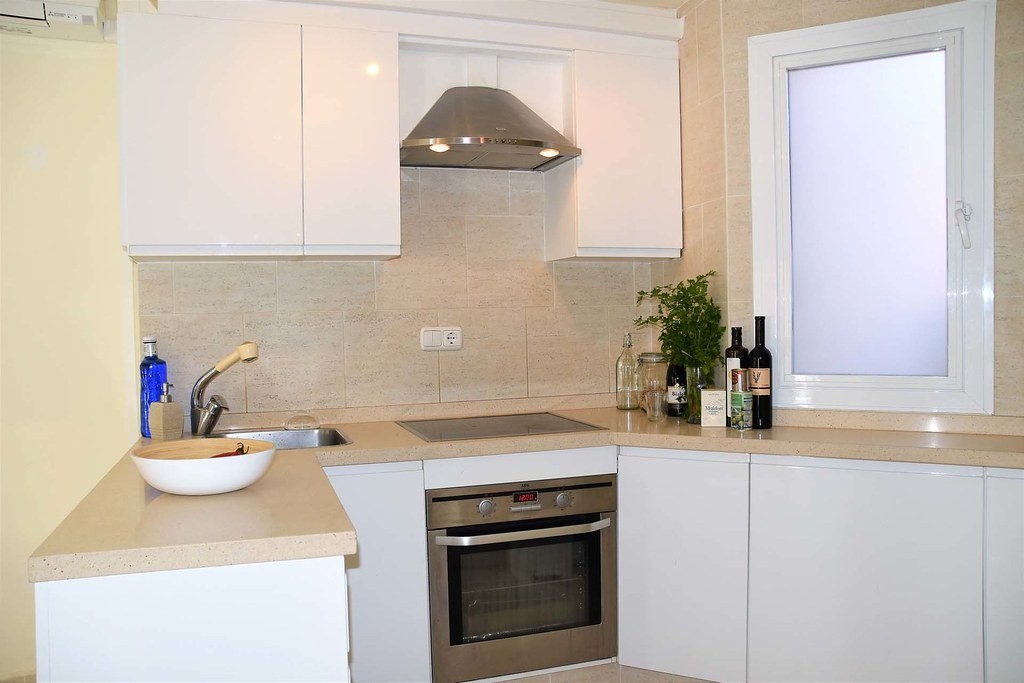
[645,391,667,422]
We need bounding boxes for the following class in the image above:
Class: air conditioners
[0,0,104,43]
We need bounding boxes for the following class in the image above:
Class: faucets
[189,341,261,437]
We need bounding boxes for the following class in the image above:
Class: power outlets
[420,326,462,351]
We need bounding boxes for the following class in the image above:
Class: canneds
[729,368,753,431]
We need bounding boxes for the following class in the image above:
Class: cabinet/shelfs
[615,455,1023,682]
[575,51,683,257]
[118,13,401,259]
[332,460,431,683]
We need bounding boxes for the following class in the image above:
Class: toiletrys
[138,336,168,438]
[150,382,185,440]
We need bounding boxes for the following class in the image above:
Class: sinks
[205,428,355,451]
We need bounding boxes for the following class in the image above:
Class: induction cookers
[395,411,609,443]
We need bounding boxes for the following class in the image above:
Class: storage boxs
[699,389,727,427]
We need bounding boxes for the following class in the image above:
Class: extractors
[398,84,582,172]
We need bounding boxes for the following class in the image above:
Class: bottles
[615,334,638,409]
[731,368,749,392]
[748,315,773,429]
[730,390,753,430]
[140,335,167,436]
[724,327,749,428]
[634,351,668,410]
[664,362,688,418]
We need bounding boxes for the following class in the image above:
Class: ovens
[425,475,619,683]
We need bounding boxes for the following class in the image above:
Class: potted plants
[632,270,726,424]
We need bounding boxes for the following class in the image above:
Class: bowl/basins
[130,438,276,495]
